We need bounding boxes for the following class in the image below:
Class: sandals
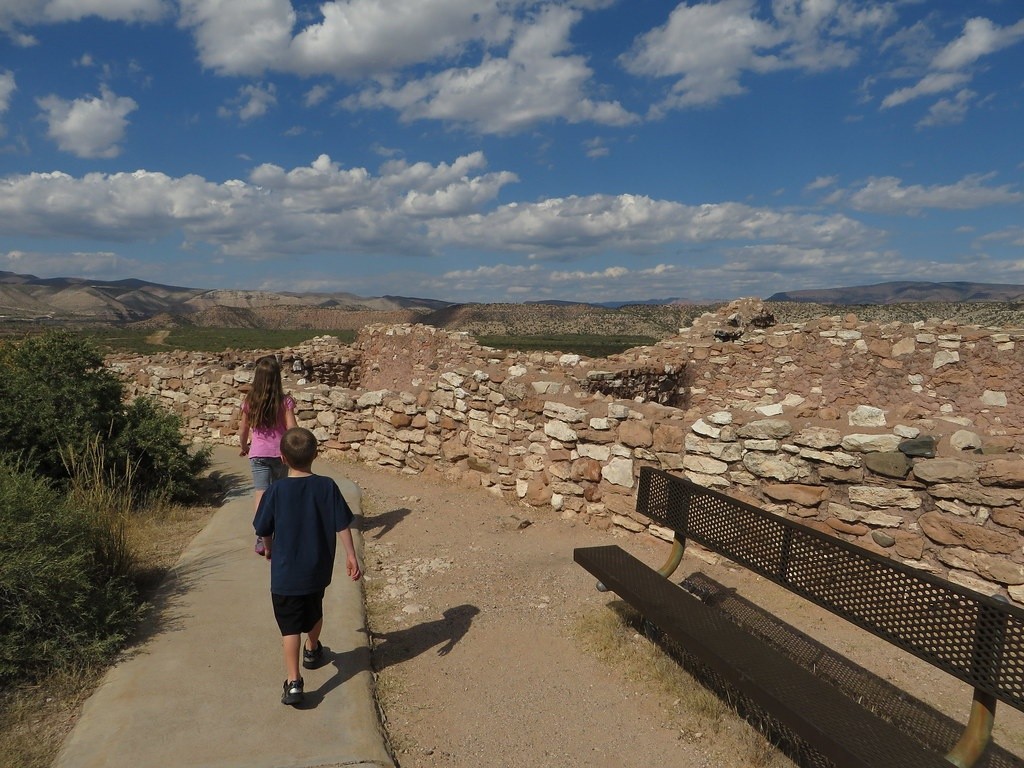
[280,677,304,704]
[302,639,323,669]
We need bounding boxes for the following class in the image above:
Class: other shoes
[255,537,265,553]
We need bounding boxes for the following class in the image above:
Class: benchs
[574,464,1024,768]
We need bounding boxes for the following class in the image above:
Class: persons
[253,428,360,704]
[239,356,299,554]
